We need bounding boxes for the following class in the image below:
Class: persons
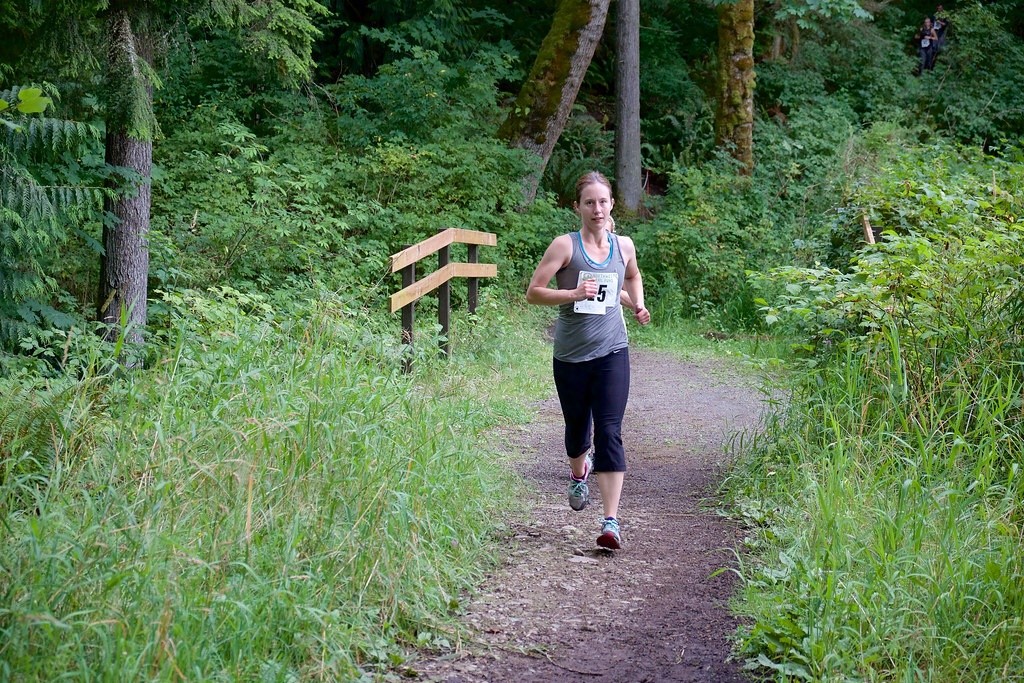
[933,3,949,39]
[914,16,938,75]
[602,214,637,308]
[526,172,652,550]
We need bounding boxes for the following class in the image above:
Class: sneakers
[596,520,623,549]
[567,456,592,511]
